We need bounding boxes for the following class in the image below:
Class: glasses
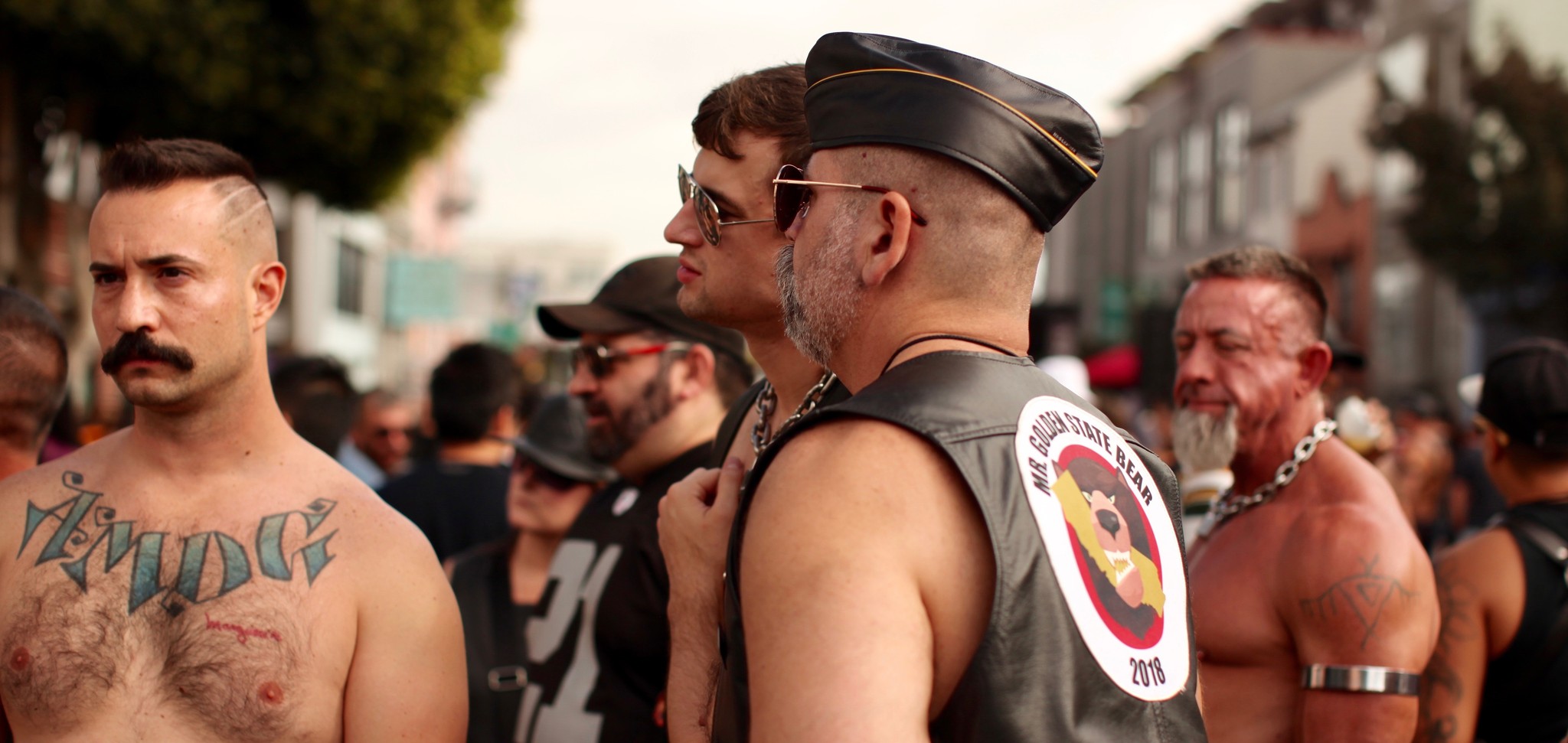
[676,162,773,247]
[773,162,928,230]
[511,452,595,493]
[569,342,689,378]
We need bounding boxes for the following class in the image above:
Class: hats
[515,394,602,482]
[802,32,1104,232]
[1034,355,1092,405]
[536,258,747,356]
[1473,341,1568,453]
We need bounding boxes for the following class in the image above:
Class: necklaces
[1197,417,1337,538]
[738,364,836,492]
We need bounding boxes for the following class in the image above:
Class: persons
[513,256,755,743]
[725,30,1210,743]
[0,139,470,743]
[656,63,854,743]
[1171,244,1444,743]
[0,30,1568,743]
